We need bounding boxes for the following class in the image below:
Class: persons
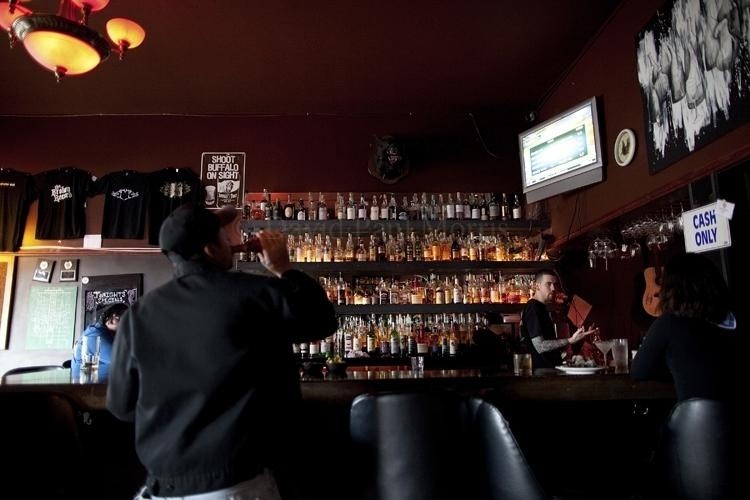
[71,303,129,368]
[630,251,749,500]
[102,203,337,500]
[521,269,599,375]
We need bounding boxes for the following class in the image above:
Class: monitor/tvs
[514,96,606,206]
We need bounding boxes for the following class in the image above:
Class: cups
[513,354,532,375]
[412,357,424,370]
[72,335,101,369]
[611,339,628,368]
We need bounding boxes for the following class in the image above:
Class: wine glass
[619,201,688,246]
[595,341,612,369]
[588,234,617,270]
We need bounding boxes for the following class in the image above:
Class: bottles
[288,228,534,261]
[319,272,534,305]
[294,311,489,357]
[240,228,262,262]
[244,190,524,222]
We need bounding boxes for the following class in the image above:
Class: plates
[555,364,603,374]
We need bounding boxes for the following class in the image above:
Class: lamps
[0,0,146,87]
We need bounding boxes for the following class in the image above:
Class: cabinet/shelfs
[237,217,551,373]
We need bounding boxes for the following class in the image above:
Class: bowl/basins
[327,362,347,375]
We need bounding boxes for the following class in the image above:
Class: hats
[160,204,235,260]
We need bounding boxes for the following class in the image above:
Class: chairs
[344,393,540,500]
